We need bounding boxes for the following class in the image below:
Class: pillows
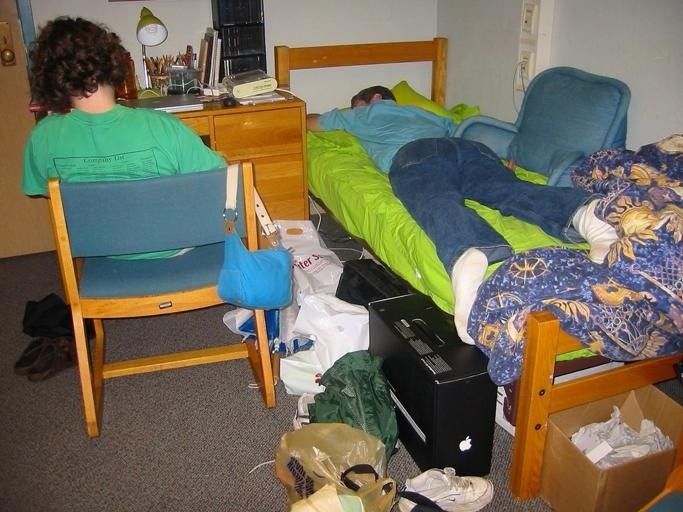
[314,80,458,149]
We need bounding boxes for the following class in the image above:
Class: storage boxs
[541,371,682,512]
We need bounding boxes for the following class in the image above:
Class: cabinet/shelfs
[211,1,268,83]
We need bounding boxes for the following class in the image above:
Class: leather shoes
[29,338,69,381]
[13,338,51,375]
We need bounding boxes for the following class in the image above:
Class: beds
[271,36,683,499]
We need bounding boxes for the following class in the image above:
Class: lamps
[134,6,170,97]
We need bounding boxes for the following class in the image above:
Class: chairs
[47,162,279,441]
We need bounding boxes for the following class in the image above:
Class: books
[196,25,222,89]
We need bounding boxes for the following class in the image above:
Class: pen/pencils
[147,45,197,76]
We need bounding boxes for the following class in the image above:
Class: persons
[21,14,232,261]
[303,83,618,346]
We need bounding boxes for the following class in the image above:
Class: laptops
[116,93,203,113]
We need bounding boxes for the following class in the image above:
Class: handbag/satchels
[279,294,369,396]
[276,220,344,306]
[275,423,386,512]
[217,163,293,311]
[290,478,397,512]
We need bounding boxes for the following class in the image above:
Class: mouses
[223,97,236,107]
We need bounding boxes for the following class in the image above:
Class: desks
[30,89,310,248]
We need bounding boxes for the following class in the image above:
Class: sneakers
[292,392,401,455]
[398,467,493,512]
